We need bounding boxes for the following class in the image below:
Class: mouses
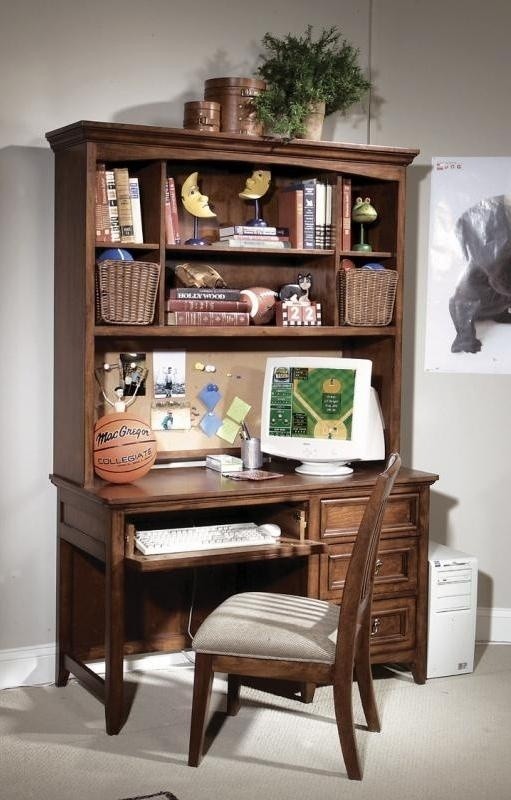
[258,523,282,537]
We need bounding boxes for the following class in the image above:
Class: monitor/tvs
[259,355,373,477]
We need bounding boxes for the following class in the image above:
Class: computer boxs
[426,540,479,680]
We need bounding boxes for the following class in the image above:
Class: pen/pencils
[239,422,251,440]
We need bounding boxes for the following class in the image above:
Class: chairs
[187,451,405,780]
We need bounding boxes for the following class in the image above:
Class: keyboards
[134,521,276,556]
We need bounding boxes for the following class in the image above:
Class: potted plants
[245,24,372,143]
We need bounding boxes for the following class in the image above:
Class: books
[165,178,181,245]
[95,164,144,244]
[211,177,332,249]
[342,178,352,250]
[164,287,252,325]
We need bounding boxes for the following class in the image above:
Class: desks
[48,464,440,738]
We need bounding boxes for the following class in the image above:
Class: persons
[164,366,173,398]
[160,410,174,431]
[96,362,147,414]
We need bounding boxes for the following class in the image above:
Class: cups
[241,437,263,469]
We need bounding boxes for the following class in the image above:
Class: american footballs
[240,287,278,324]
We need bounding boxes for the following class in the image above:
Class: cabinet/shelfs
[45,120,422,489]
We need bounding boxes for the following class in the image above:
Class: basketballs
[94,413,157,483]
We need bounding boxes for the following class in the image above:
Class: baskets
[94,259,161,325]
[338,268,399,326]
